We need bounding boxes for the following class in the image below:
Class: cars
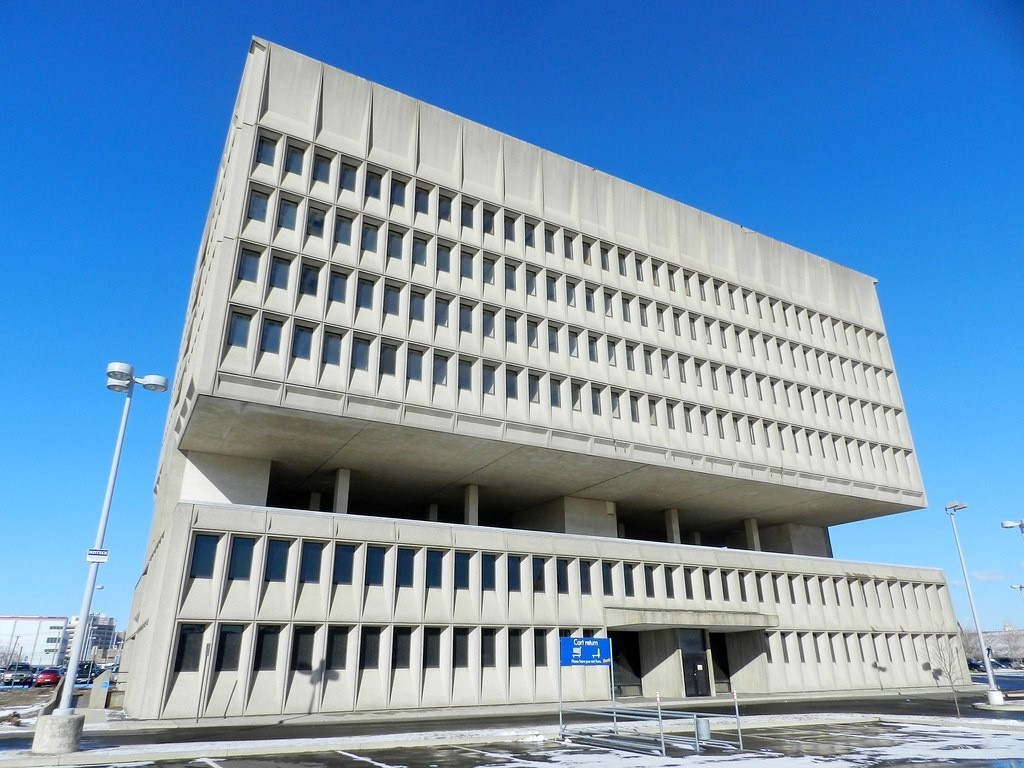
[75,660,100,684]
[966,657,984,671]
[980,661,1006,669]
[110,666,119,683]
[1006,662,1024,669]
[3,662,33,684]
[35,668,63,687]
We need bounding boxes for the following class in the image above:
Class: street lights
[53,360,168,717]
[944,501,1006,706]
